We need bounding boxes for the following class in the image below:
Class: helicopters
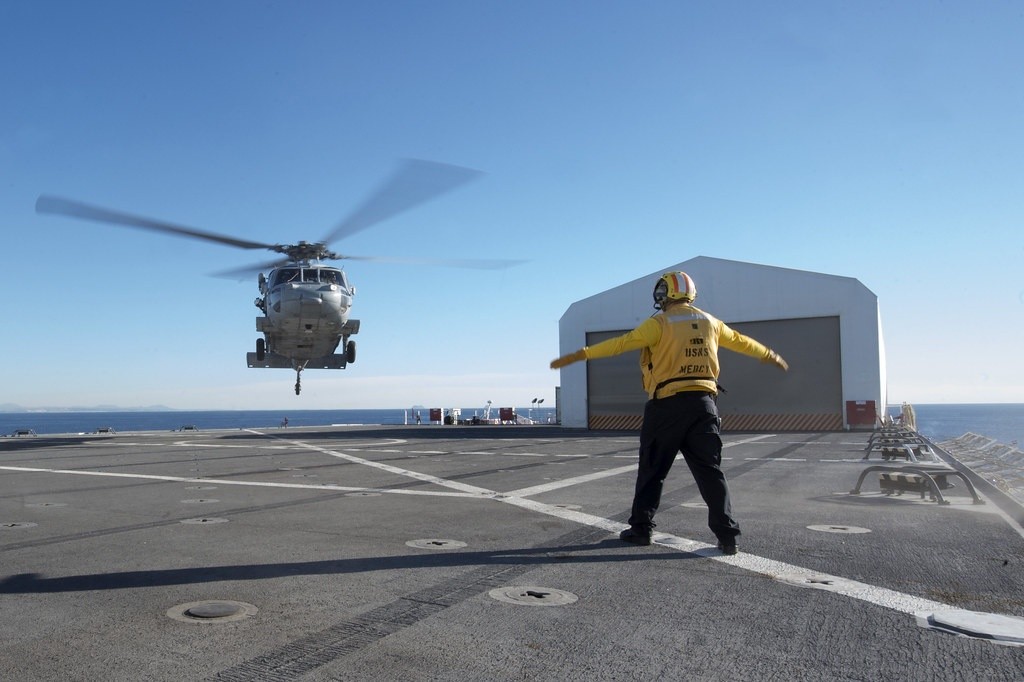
[33,153,529,396]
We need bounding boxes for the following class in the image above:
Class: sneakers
[620,529,651,546]
[717,539,738,555]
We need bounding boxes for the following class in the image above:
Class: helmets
[652,271,697,310]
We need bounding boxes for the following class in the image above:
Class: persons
[551,271,792,556]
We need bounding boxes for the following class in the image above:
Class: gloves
[550,349,586,369]
[764,349,789,372]
